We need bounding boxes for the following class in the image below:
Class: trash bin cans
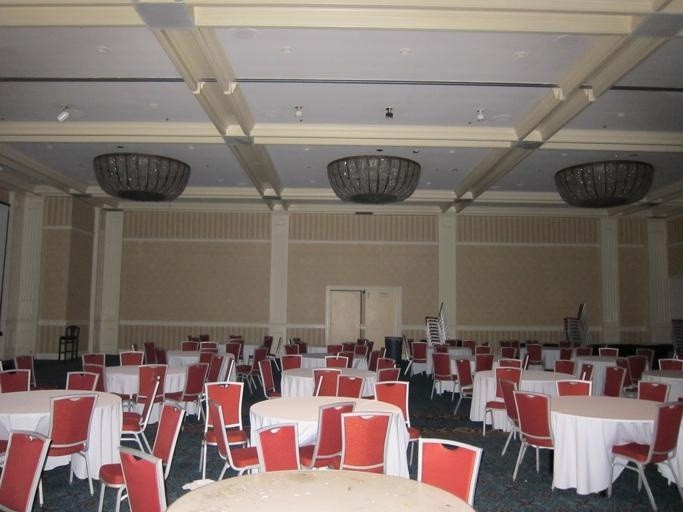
[384,336,403,361]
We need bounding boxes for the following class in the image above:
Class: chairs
[0,334,682,511]
[563,303,587,345]
[57,324,82,364]
[423,301,448,347]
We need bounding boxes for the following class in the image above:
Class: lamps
[292,104,305,123]
[55,103,71,124]
[475,107,485,122]
[385,107,395,121]
[552,156,658,210]
[551,87,568,102]
[579,85,597,104]
[89,148,193,206]
[323,152,423,209]
